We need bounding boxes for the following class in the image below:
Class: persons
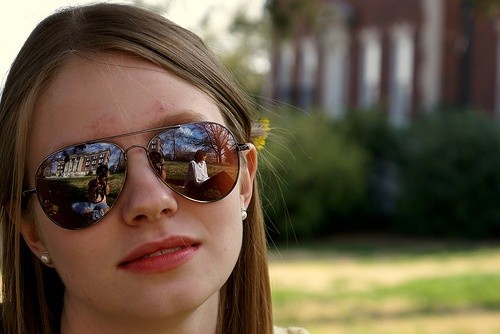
[0,0,309,333]
[148,151,167,179]
[72,165,111,219]
[184,150,235,197]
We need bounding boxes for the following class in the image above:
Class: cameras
[99,171,107,177]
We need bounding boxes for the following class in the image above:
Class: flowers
[249,117,270,150]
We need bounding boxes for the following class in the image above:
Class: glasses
[21,121,251,231]
